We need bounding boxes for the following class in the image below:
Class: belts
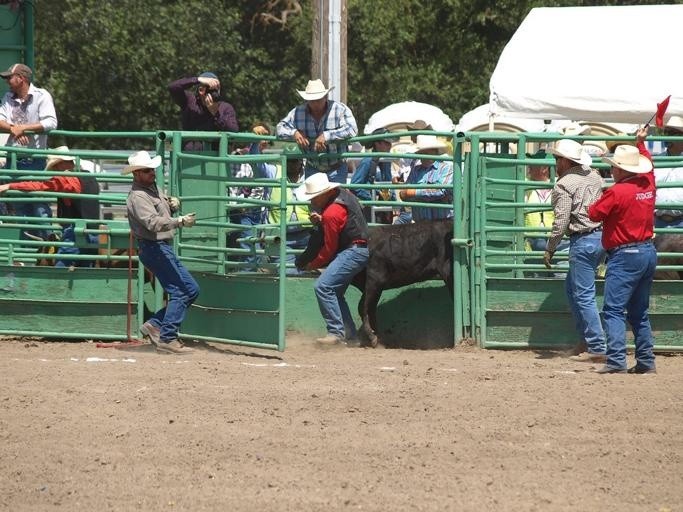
[609,239,655,253]
[570,226,604,235]
[137,239,168,245]
[345,242,369,249]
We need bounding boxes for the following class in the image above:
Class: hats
[546,139,593,166]
[292,171,341,201]
[601,145,654,174]
[664,114,683,131]
[43,144,75,170]
[120,149,162,174]
[370,125,399,143]
[526,148,551,163]
[296,78,335,101]
[1,63,31,82]
[405,134,446,153]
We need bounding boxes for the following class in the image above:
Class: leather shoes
[315,333,346,346]
[593,365,628,374]
[157,335,195,355]
[569,349,606,363]
[568,339,598,354]
[140,320,161,347]
[626,365,658,375]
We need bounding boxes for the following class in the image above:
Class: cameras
[204,82,220,103]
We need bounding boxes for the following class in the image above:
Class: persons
[167,72,239,155]
[0,63,57,265]
[543,138,609,363]
[294,172,371,349]
[277,79,360,184]
[656,115,683,229]
[587,126,658,376]
[349,127,401,220]
[391,131,455,222]
[233,123,278,271]
[524,150,568,252]
[0,144,101,267]
[248,126,317,277]
[122,149,200,357]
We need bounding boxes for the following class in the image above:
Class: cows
[293,215,453,347]
[653,227,682,281]
